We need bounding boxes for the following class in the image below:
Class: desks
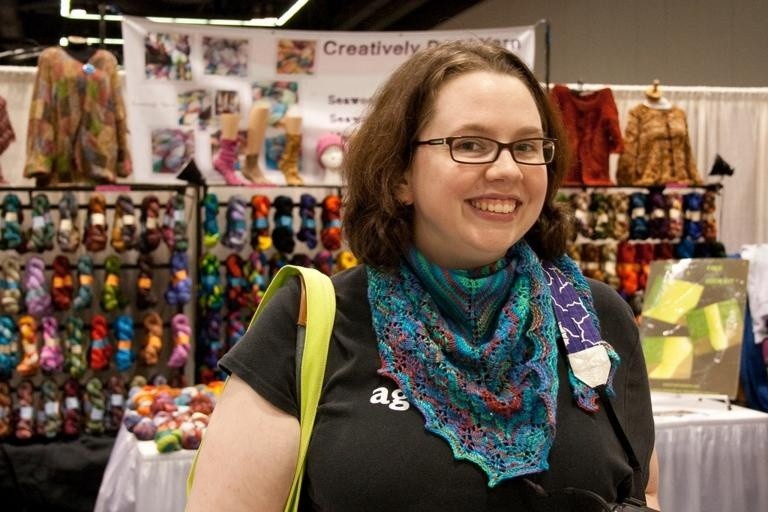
[95,390,767,512]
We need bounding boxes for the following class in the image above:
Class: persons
[614,79,702,185]
[184,41,661,510]
[212,104,308,188]
[316,132,346,184]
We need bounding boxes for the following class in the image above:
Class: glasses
[418,136,558,165]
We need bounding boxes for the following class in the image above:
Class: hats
[317,134,345,169]
